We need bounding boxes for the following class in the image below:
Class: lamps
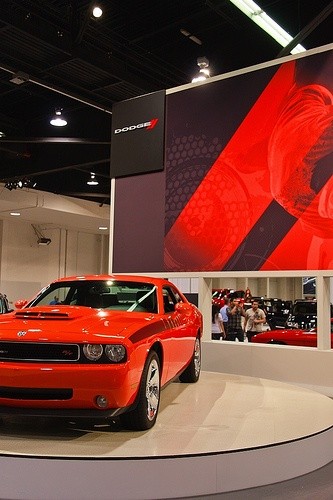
[4,177,37,192]
[49,107,69,127]
[192,56,210,84]
[86,173,99,185]
[30,224,52,246]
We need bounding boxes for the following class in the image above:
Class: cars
[0,274,204,432]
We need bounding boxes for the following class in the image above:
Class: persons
[0,293,9,314]
[212,296,266,342]
[49,296,61,305]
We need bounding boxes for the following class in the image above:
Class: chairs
[75,288,169,311]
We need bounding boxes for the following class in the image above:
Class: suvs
[211,288,333,348]
